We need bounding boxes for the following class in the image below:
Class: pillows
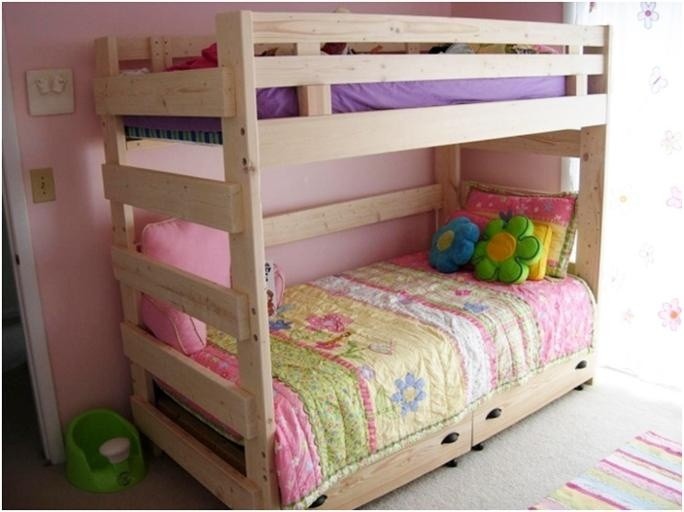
[470,215,543,284]
[460,179,579,278]
[141,218,215,355]
[429,43,561,53]
[456,207,553,282]
[430,216,480,273]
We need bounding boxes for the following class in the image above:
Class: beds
[94,9,613,512]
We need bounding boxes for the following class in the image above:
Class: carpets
[523,428,682,510]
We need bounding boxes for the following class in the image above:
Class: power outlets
[30,167,56,204]
